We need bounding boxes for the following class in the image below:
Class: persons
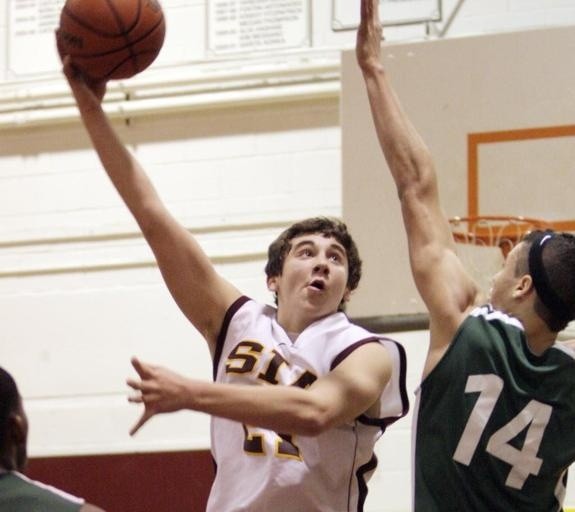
[356,1,574,512]
[55,30,394,512]
[0,368,105,512]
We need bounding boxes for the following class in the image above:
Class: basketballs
[59,1,165,79]
